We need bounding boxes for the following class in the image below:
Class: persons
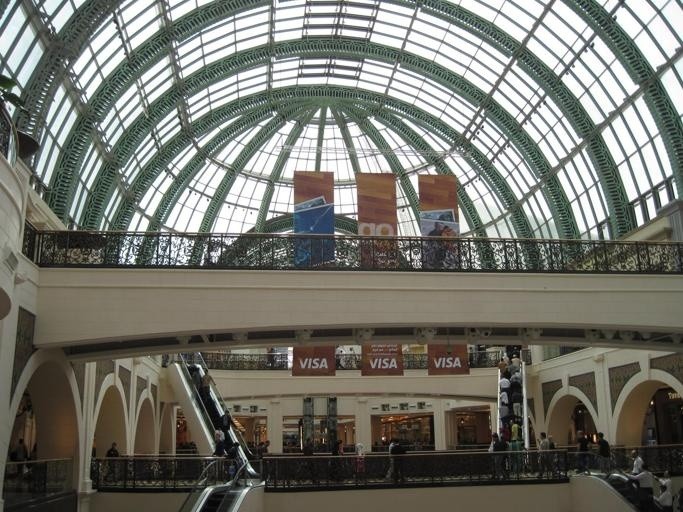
[11,438,29,481]
[31,442,39,460]
[179,347,411,480]
[466,344,683,512]
[103,442,120,483]
